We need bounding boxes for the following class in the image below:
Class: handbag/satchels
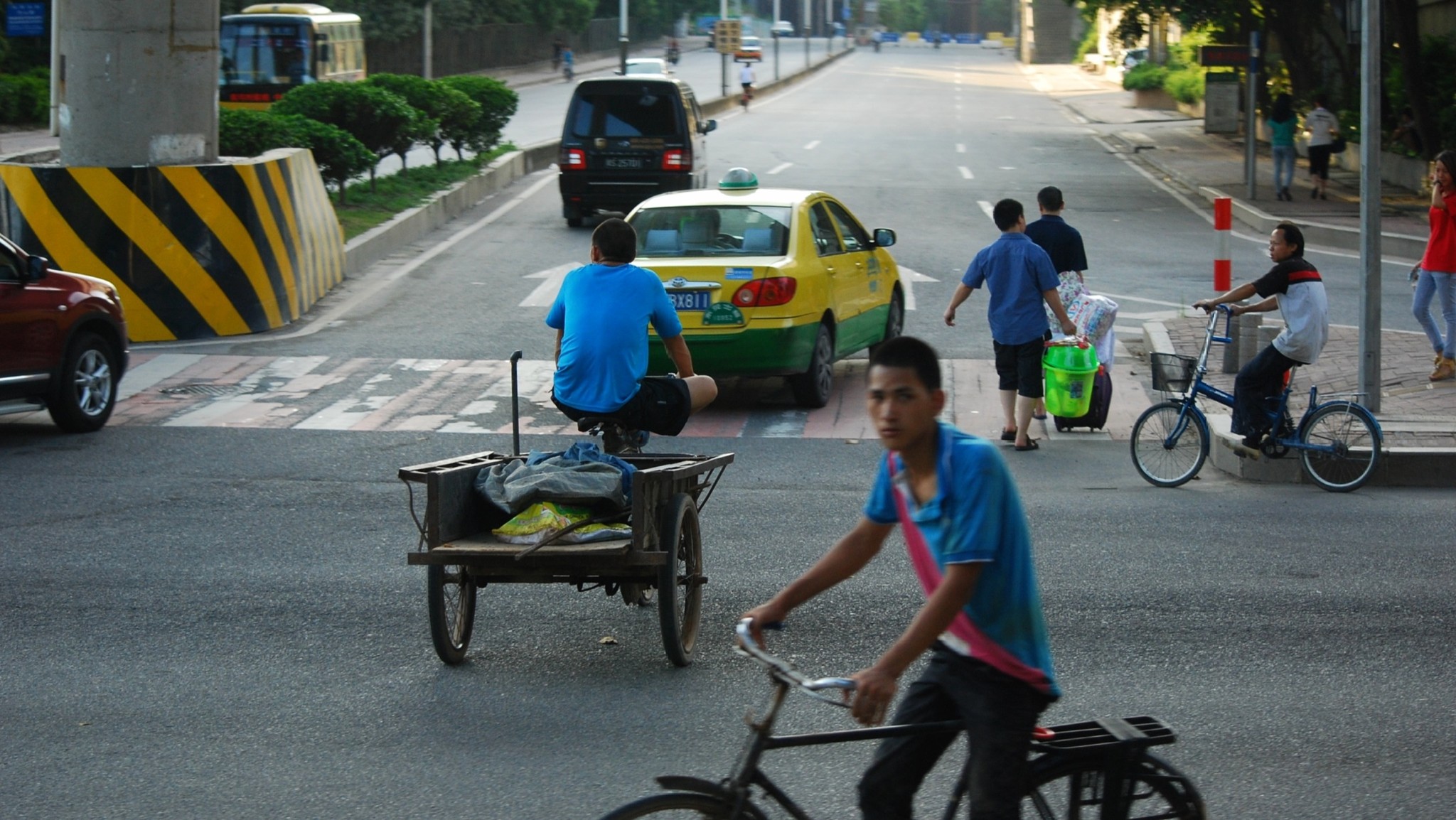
[1331,136,1347,154]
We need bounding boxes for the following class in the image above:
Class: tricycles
[397,349,735,671]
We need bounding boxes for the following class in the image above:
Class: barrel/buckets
[1041,331,1100,418]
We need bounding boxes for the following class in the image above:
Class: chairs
[682,221,718,249]
[645,229,680,251]
[742,229,775,250]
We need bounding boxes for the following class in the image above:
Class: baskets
[1149,351,1198,393]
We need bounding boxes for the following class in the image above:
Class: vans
[558,77,717,228]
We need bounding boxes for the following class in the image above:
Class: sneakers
[1221,437,1261,461]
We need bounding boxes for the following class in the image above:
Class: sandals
[1434,353,1443,367]
[1428,357,1456,380]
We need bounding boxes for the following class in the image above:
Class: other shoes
[1282,187,1292,201]
[1312,187,1318,199]
[1277,192,1284,201]
[1320,194,1327,200]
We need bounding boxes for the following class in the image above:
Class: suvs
[0,231,132,434]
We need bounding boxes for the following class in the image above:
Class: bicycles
[595,616,1209,820]
[1128,302,1385,493]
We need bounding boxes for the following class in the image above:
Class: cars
[734,37,765,62]
[614,57,675,77]
[769,21,795,38]
[622,167,906,413]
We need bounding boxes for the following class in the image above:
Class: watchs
[1434,179,1442,187]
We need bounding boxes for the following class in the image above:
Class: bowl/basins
[1043,342,1100,370]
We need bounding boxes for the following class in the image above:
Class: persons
[1267,95,1298,201]
[944,185,1087,450]
[1304,94,1341,198]
[738,335,1060,820]
[551,36,757,107]
[1387,108,1424,159]
[933,31,942,49]
[871,29,882,52]
[544,217,719,437]
[1412,150,1456,381]
[1191,221,1330,448]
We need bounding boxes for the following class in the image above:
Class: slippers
[1032,411,1047,419]
[1001,426,1018,442]
[1015,434,1039,450]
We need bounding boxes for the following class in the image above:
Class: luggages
[1053,367,1112,433]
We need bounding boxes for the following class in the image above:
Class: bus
[218,3,367,112]
